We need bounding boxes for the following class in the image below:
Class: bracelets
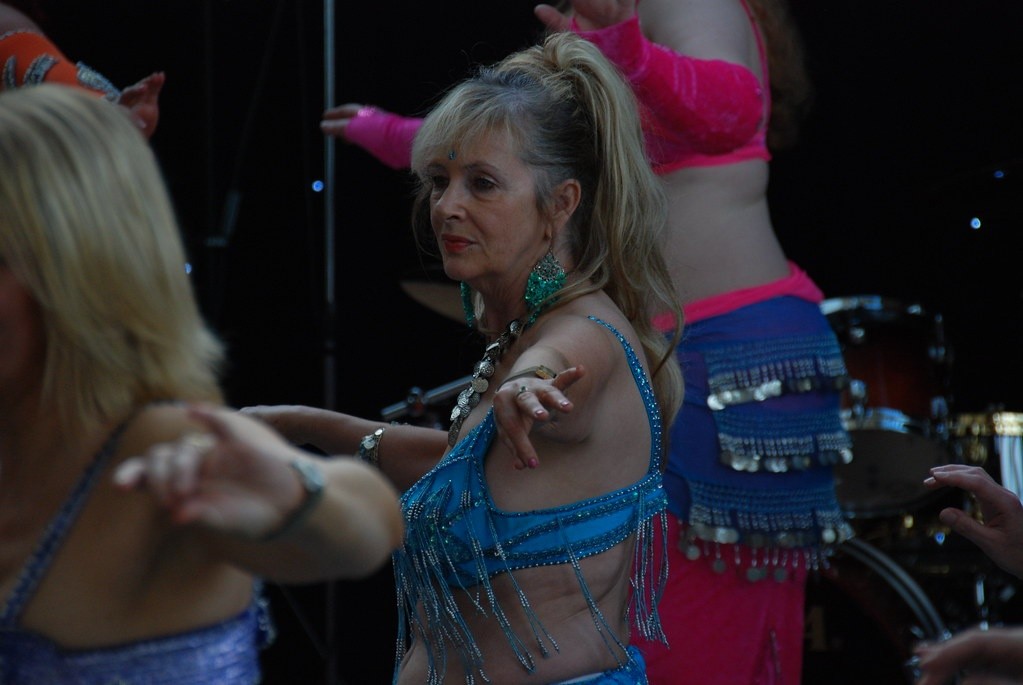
[362,420,400,466]
[260,461,326,544]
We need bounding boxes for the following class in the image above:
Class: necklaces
[450,317,521,445]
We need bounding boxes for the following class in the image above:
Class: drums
[801,515,1004,685]
[818,294,956,520]
[856,411,1023,542]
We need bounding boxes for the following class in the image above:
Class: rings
[515,386,531,398]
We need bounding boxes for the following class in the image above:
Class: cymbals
[399,278,477,328]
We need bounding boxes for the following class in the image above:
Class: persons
[922,463,1023,685]
[320,0,851,684]
[0,2,164,139]
[0,83,404,684]
[237,31,670,685]
